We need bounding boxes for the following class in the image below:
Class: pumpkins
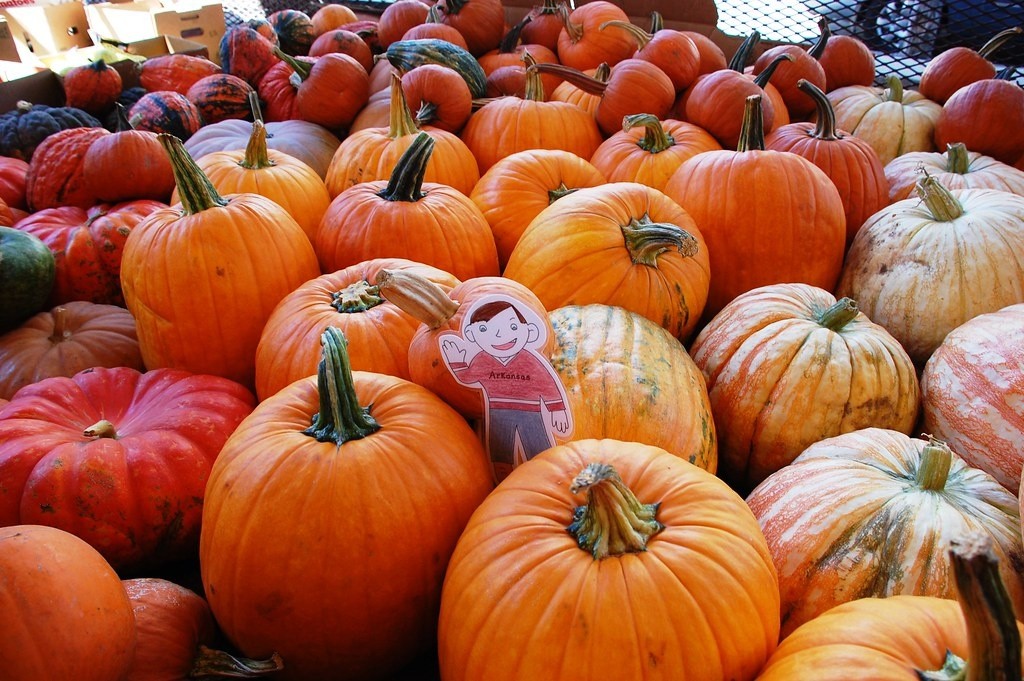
[0,0,1024,681]
